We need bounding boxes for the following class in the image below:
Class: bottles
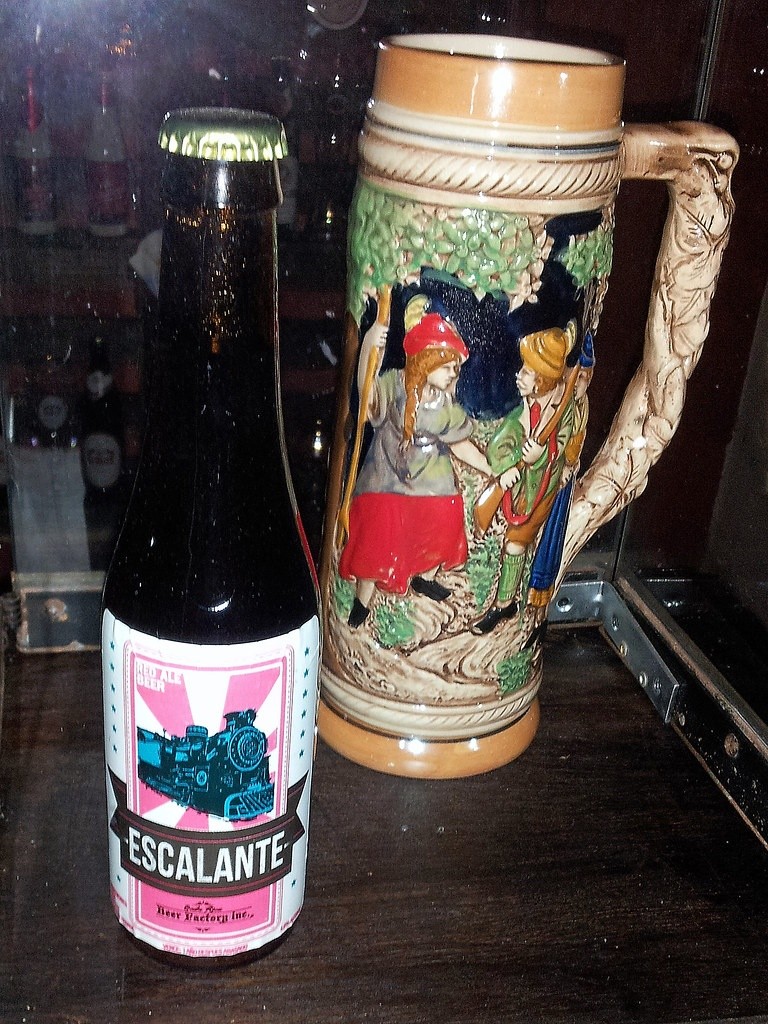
[86,78,130,236]
[13,64,55,235]
[100,107,324,957]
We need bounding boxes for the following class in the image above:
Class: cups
[319,33,740,780]
